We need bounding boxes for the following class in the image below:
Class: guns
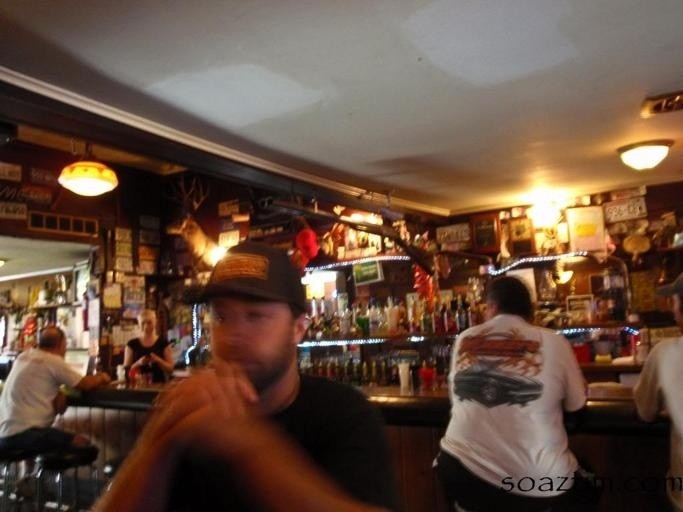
[259,198,435,275]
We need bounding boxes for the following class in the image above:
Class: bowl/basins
[592,340,616,354]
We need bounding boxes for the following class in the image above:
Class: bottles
[311,358,420,386]
[308,293,481,340]
[143,352,153,387]
[37,274,74,304]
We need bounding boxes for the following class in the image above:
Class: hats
[181,240,305,308]
[654,272,683,297]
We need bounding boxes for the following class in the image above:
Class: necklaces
[269,378,299,418]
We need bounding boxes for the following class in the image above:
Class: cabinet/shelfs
[0,262,104,373]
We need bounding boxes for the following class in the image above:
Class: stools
[33,446,99,512]
[1,450,44,509]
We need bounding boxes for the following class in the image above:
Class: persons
[633,271,683,512]
[88,242,393,512]
[123,310,175,385]
[431,276,589,511]
[0,326,111,506]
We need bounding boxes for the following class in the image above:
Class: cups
[573,343,593,363]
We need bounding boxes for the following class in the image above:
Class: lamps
[57,138,120,199]
[616,138,674,171]
